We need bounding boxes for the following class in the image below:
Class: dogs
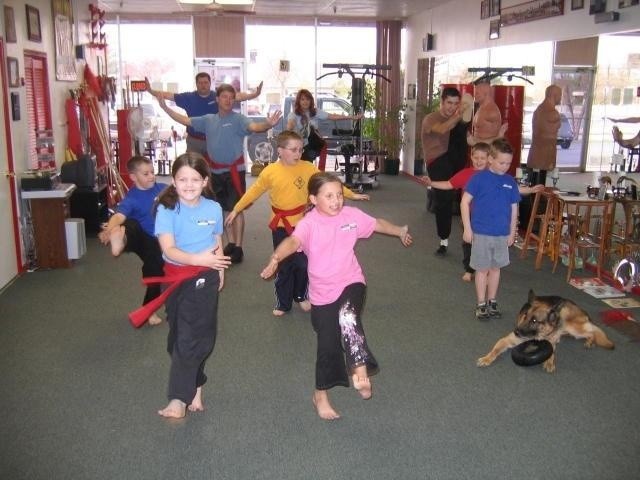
[477,288,615,373]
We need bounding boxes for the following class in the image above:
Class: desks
[608,193,640,263]
[552,196,614,283]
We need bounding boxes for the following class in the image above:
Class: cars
[109,101,175,147]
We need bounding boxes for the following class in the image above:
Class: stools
[520,187,561,269]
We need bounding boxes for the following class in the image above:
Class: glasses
[282,147,305,153]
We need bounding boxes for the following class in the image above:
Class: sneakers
[223,244,243,264]
[474,302,489,320]
[487,300,501,317]
[436,245,447,257]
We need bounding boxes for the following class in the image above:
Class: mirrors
[414,28,640,294]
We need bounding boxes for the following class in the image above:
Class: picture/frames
[481,0,584,40]
[7,57,19,87]
[26,4,43,43]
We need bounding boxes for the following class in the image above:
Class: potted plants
[363,97,414,175]
[414,91,440,175]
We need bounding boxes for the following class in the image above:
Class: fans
[127,104,162,184]
[171,0,257,17]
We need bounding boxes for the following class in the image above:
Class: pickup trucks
[250,92,392,165]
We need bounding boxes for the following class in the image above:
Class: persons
[223,130,371,316]
[156,83,284,261]
[286,89,364,162]
[420,86,509,257]
[421,142,546,282]
[96,155,168,325]
[145,72,264,155]
[129,151,232,419]
[525,85,563,169]
[461,137,522,320]
[472,80,502,139]
[260,171,414,420]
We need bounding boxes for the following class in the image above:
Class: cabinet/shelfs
[21,183,77,270]
[71,184,108,238]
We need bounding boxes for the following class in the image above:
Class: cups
[598,185,606,201]
[587,187,596,199]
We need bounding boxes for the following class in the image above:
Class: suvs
[518,106,573,148]
